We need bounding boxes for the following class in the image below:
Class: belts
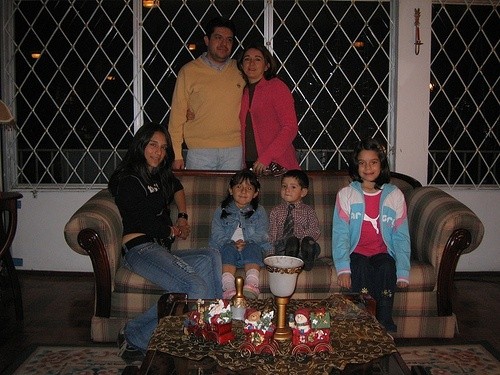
[121,235,171,257]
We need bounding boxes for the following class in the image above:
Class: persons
[108,123,224,365]
[209,169,272,299]
[168,16,249,171]
[237,44,302,177]
[269,169,321,271]
[332,139,411,332]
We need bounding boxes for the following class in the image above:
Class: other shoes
[222,288,236,300]
[301,236,315,271]
[285,236,299,258]
[118,326,145,366]
[383,320,397,332]
[243,283,260,299]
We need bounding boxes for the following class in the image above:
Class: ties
[282,204,295,246]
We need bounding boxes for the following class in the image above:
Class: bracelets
[177,213,188,220]
[169,226,175,240]
[173,225,182,237]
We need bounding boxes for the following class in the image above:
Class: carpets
[11,344,500,375]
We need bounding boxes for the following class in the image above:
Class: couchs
[65,169,484,343]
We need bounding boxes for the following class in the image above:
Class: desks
[121,292,427,375]
